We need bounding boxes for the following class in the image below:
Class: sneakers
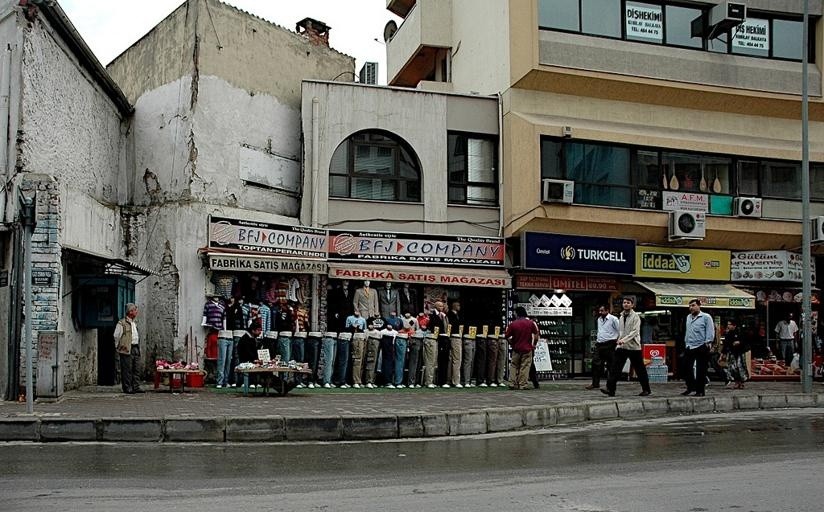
[681,388,695,396]
[518,382,531,390]
[600,387,617,397]
[726,381,745,389]
[639,389,652,397]
[215,380,506,390]
[122,387,146,394]
[585,382,600,389]
[691,391,705,397]
[508,381,517,389]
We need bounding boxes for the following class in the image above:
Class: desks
[234,363,313,398]
[154,366,199,394]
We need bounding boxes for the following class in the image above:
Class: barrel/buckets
[186,373,204,388]
[207,332,217,357]
[172,373,182,388]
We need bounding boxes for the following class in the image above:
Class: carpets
[207,382,514,396]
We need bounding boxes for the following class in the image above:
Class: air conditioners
[540,177,576,204]
[733,196,764,220]
[668,208,709,241]
[811,215,824,244]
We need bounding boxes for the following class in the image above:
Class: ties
[365,289,369,298]
[386,290,391,300]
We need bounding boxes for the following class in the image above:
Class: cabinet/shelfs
[524,312,575,383]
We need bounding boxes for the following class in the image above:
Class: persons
[585,304,620,390]
[526,317,542,387]
[680,300,715,396]
[113,302,146,394]
[774,312,799,359]
[200,276,508,389]
[599,297,651,397]
[504,306,539,390]
[717,319,748,390]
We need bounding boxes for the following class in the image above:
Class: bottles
[645,357,668,385]
